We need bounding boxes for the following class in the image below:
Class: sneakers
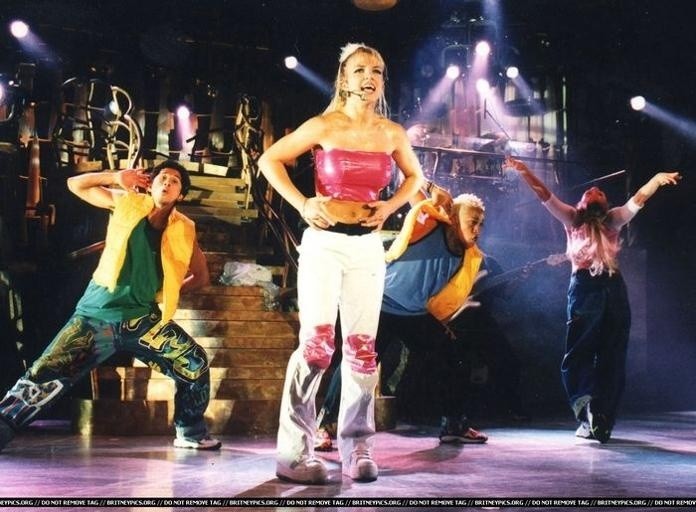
[341,446,379,481]
[439,421,488,445]
[586,395,610,444]
[313,428,333,452]
[173,431,221,452]
[576,419,594,438]
[276,451,328,483]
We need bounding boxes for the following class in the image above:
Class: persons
[500,154,685,444]
[256,42,426,485]
[0,158,224,452]
[448,253,534,430]
[311,167,492,453]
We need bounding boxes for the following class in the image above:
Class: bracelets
[426,180,435,195]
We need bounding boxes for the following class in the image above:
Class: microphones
[483,97,486,120]
[346,89,370,101]
